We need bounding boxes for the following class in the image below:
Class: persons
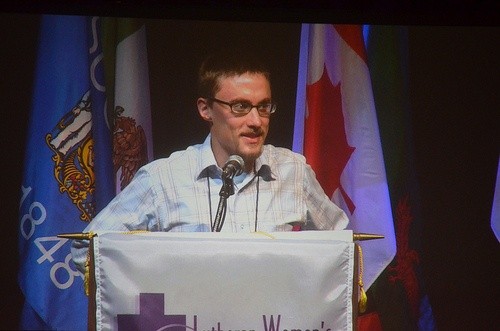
[66,47,349,275]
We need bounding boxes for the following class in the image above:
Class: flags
[295,24,397,310]
[16,12,159,331]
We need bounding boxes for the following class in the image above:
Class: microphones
[221,155,245,180]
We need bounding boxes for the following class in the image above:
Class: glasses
[206,96,278,116]
